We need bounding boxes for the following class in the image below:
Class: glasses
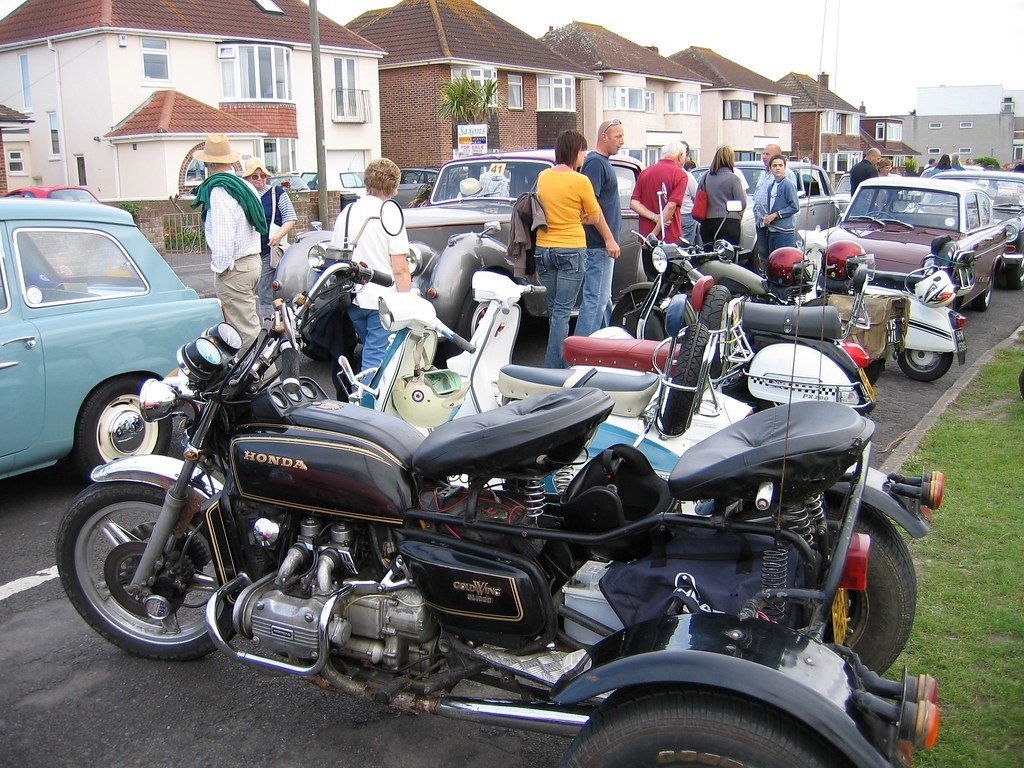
[251,174,267,180]
[604,119,622,134]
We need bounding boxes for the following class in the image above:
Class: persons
[751,144,797,273]
[697,145,750,253]
[682,142,698,245]
[764,155,798,252]
[575,121,624,338]
[684,162,696,171]
[629,142,688,281]
[194,134,278,387]
[242,159,297,316]
[850,148,901,210]
[924,159,935,171]
[329,157,411,409]
[932,154,964,174]
[535,131,620,370]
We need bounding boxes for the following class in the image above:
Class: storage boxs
[747,339,876,412]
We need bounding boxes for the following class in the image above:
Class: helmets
[558,443,678,564]
[953,268,975,297]
[915,271,956,308]
[820,241,867,281]
[765,247,809,287]
[392,364,471,428]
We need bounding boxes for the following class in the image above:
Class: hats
[457,178,485,200]
[242,157,271,179]
[193,134,240,163]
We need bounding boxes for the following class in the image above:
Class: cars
[687,160,841,244]
[0,196,227,493]
[834,173,895,212]
[266,169,368,199]
[2,185,100,202]
[903,170,1023,291]
[819,175,1006,315]
[273,149,651,369]
[389,166,440,207]
[919,165,986,179]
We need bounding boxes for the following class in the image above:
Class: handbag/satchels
[269,243,290,268]
[692,171,710,223]
[517,176,537,225]
[310,296,356,360]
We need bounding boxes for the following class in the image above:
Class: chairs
[888,211,958,230]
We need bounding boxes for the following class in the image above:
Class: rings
[652,214,655,221]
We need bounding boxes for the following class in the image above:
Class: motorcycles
[55,197,947,768]
[335,179,976,519]
